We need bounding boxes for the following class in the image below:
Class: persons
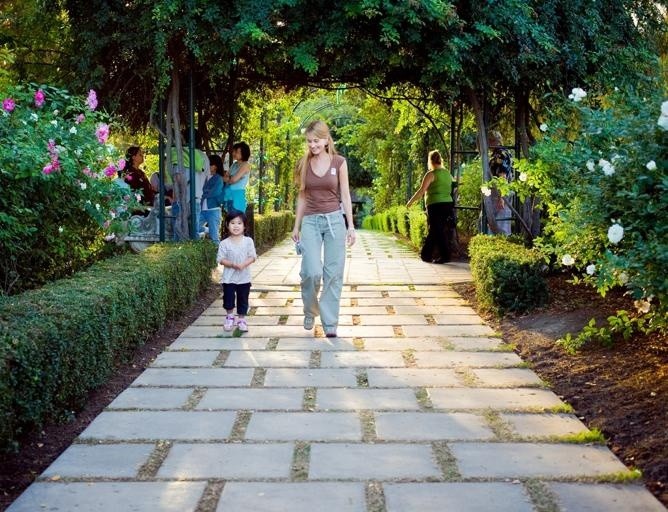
[292,121,356,337]
[405,148,457,265]
[216,211,257,331]
[477,130,515,235]
[117,127,250,244]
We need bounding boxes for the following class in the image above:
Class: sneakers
[325,326,336,337]
[237,317,248,332]
[223,314,235,331]
[304,314,315,330]
